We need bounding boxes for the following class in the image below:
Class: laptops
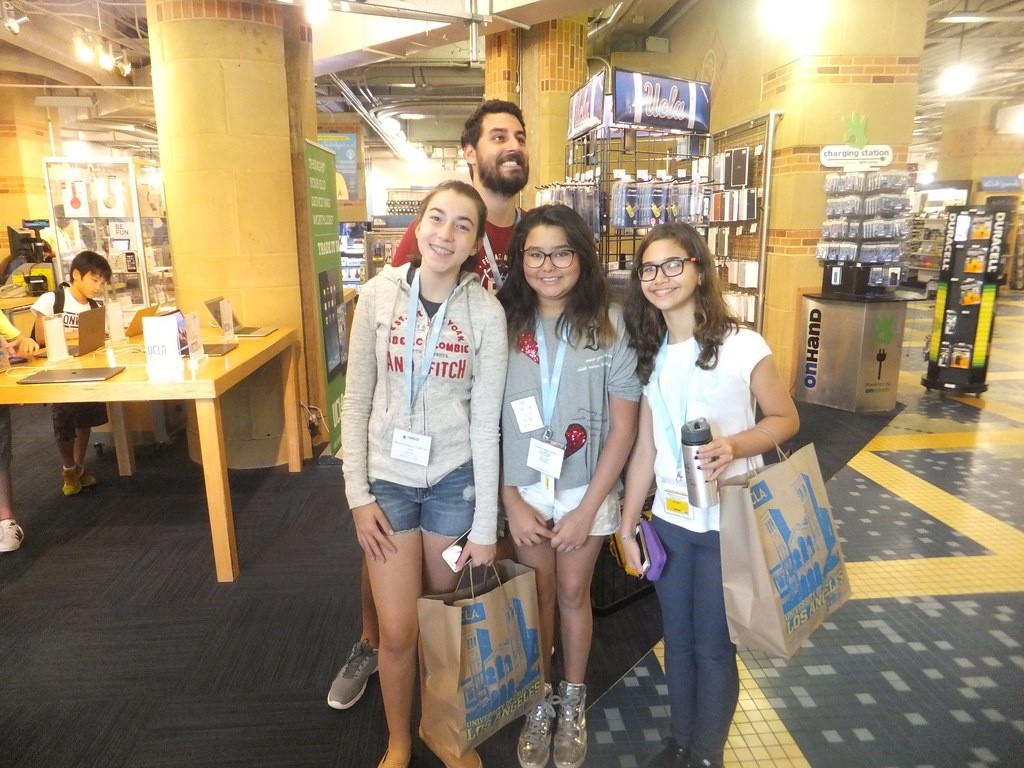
[16,367,125,383]
[105,304,160,338]
[203,296,278,337]
[33,306,106,358]
[159,309,239,357]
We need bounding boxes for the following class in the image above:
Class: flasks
[680,421,717,508]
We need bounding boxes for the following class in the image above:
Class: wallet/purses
[641,520,667,581]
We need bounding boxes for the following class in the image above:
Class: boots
[516,683,555,768]
[552,682,587,768]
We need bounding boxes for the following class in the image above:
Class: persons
[328,98,534,710]
[0,239,54,289]
[341,180,509,768]
[502,205,641,768]
[29,250,113,496]
[621,222,800,768]
[0,310,40,553]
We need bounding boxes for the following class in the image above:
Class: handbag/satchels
[718,426,852,660]
[417,559,544,759]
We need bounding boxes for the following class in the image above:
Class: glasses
[520,248,577,269]
[637,257,697,282]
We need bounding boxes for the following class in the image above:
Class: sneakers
[0,518,24,552]
[327,638,379,709]
[81,472,96,486]
[62,463,84,495]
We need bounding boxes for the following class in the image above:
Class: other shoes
[648,737,724,768]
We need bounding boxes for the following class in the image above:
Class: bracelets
[619,533,636,542]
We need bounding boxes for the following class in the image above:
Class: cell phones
[634,524,650,574]
[442,528,474,573]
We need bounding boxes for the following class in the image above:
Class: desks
[0,327,303,583]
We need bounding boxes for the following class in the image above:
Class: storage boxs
[142,306,191,363]
